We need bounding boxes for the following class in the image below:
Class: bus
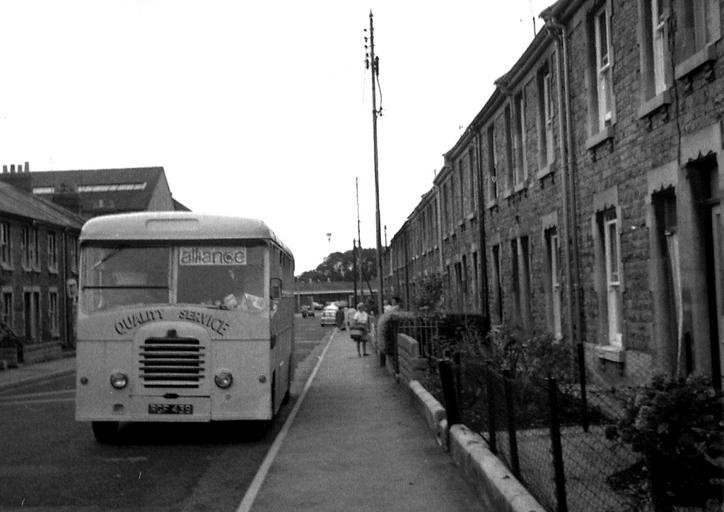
[74,211,295,443]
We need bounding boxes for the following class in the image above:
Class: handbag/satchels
[349,321,365,340]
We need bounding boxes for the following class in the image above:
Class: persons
[350,302,372,358]
[335,305,356,330]
[382,297,401,313]
[204,273,264,311]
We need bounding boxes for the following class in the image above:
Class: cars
[320,305,339,327]
